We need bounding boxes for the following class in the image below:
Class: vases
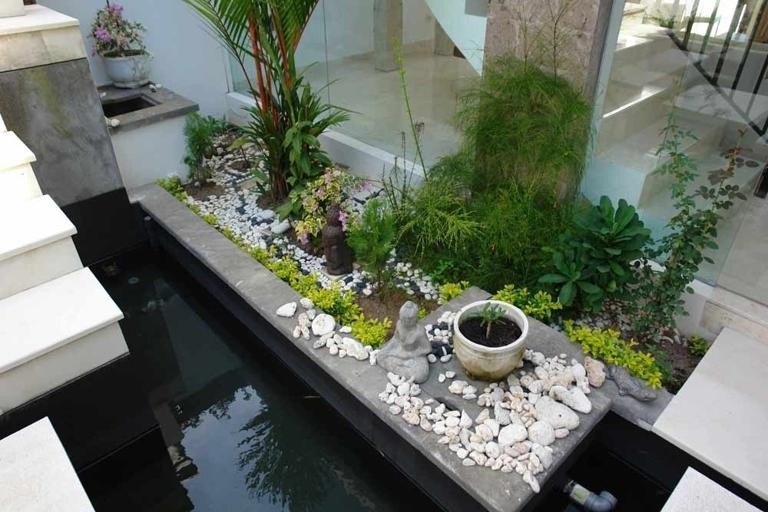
[101,48,155,90]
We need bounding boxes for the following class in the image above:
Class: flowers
[85,1,151,58]
[282,163,376,246]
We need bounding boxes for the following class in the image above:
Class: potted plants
[454,298,531,383]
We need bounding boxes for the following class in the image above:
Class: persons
[376,301,432,384]
[321,204,353,274]
